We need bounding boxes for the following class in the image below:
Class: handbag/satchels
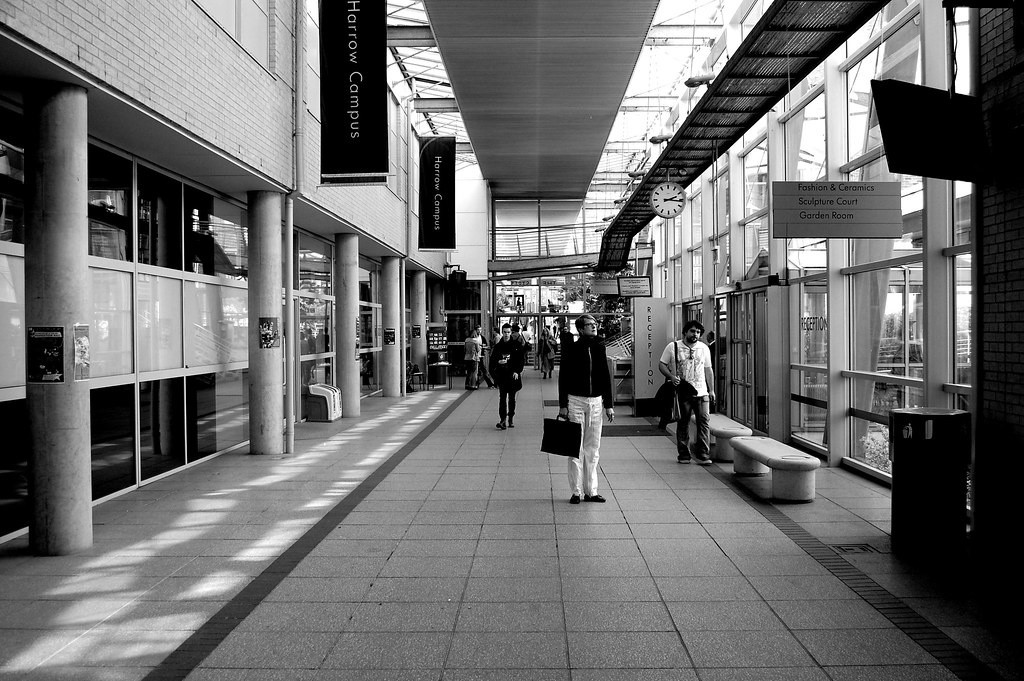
[540,414,582,460]
[661,389,681,423]
[548,335,558,353]
[524,341,532,352]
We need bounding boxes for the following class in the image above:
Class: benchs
[691,413,752,463]
[727,436,821,503]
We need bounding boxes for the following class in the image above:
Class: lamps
[684,74,716,91]
[601,215,615,222]
[650,132,674,146]
[614,197,629,205]
[594,227,607,232]
[628,169,646,183]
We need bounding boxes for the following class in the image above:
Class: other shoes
[464,386,479,391]
[697,455,712,463]
[570,493,580,504]
[677,458,690,464]
[584,494,606,502]
[488,384,497,389]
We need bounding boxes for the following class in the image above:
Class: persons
[706,331,716,414]
[658,320,716,465]
[517,298,521,311]
[521,326,531,364]
[463,324,494,390]
[558,314,615,504]
[492,327,502,344]
[561,326,574,353]
[299,327,331,385]
[491,323,525,430]
[511,325,525,347]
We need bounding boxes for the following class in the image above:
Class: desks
[427,363,453,391]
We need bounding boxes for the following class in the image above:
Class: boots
[508,417,515,427]
[496,417,506,430]
[542,372,547,379]
[549,370,552,378]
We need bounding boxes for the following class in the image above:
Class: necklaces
[536,328,557,379]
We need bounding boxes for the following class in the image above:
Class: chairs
[405,362,425,393]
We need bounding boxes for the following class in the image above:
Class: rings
[612,415,614,417]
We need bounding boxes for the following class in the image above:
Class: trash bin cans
[889,408,971,566]
[427,351,447,385]
[607,355,634,407]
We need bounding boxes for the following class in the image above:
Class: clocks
[648,183,687,220]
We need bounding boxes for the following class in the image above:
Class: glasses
[583,323,597,328]
